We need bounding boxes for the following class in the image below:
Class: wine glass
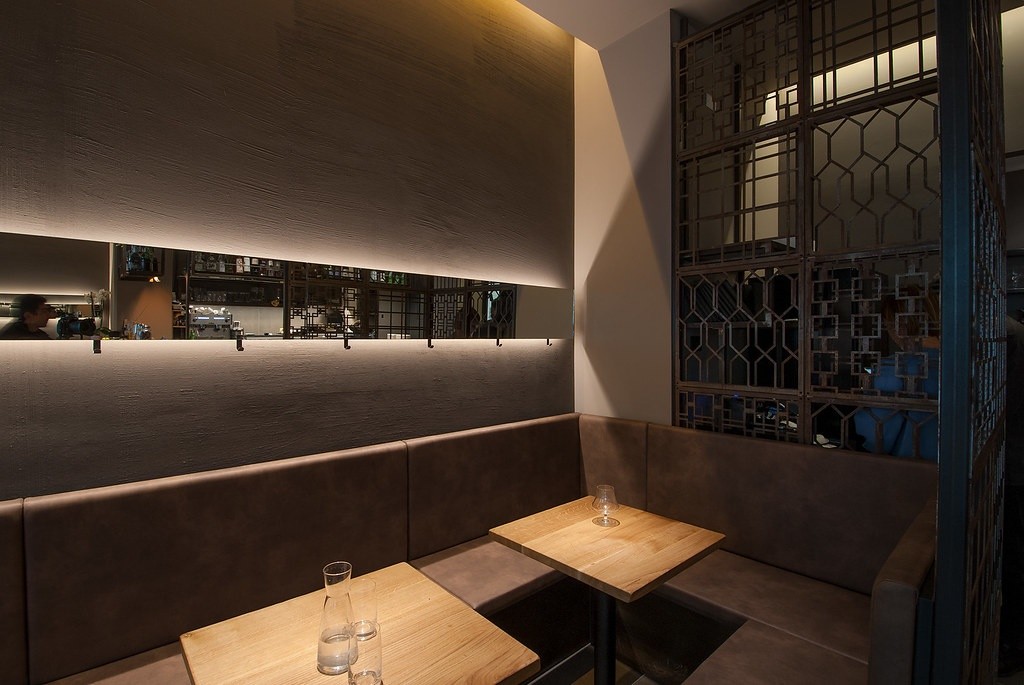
[592,485,620,527]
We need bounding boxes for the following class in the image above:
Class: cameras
[57,312,96,337]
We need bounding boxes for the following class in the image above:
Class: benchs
[0,411,937,685]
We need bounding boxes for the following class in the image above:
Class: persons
[1,294,54,340]
[449,306,480,339]
[474,297,513,339]
[856,287,1022,460]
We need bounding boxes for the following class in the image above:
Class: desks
[488,497,725,685]
[179,560,541,685]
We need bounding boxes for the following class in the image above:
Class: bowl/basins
[228,292,251,302]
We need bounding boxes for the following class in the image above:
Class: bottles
[123,319,151,340]
[192,252,226,273]
[126,245,159,273]
[317,561,352,675]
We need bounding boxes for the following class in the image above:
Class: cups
[189,287,226,301]
[346,622,383,685]
[348,579,377,638]
